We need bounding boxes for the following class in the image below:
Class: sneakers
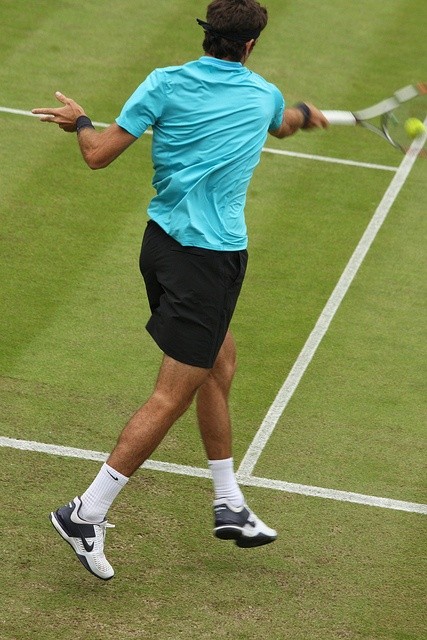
[50,497,115,581]
[213,499,279,550]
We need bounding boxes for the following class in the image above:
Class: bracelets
[296,101,312,130]
[75,114,94,135]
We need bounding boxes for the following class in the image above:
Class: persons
[30,1,331,581]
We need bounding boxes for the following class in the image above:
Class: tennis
[405,118,426,138]
